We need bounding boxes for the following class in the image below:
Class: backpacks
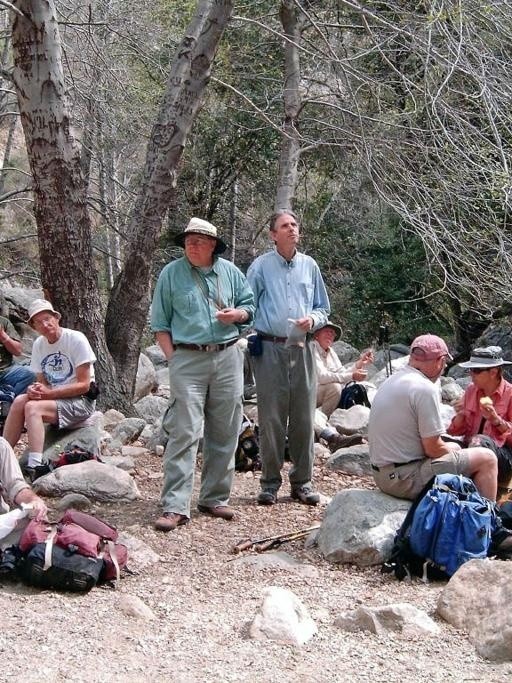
[336,380,371,410]
[0,509,128,593]
[380,472,512,583]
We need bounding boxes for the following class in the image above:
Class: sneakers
[325,434,362,452]
[290,483,320,503]
[196,498,235,520]
[21,464,53,486]
[153,508,187,530]
[256,484,279,505]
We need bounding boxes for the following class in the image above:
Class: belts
[253,331,310,344]
[170,341,239,353]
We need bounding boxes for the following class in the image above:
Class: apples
[478,395,493,406]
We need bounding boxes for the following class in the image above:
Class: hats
[313,320,342,343]
[410,334,454,361]
[457,346,512,370]
[24,298,61,326]
[173,216,229,252]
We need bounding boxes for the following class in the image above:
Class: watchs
[239,310,244,323]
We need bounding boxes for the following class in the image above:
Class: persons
[367,333,499,503]
[245,208,330,505]
[151,215,256,530]
[0,436,48,523]
[315,409,362,453]
[0,316,37,402]
[307,320,375,413]
[2,299,101,484]
[447,346,512,482]
